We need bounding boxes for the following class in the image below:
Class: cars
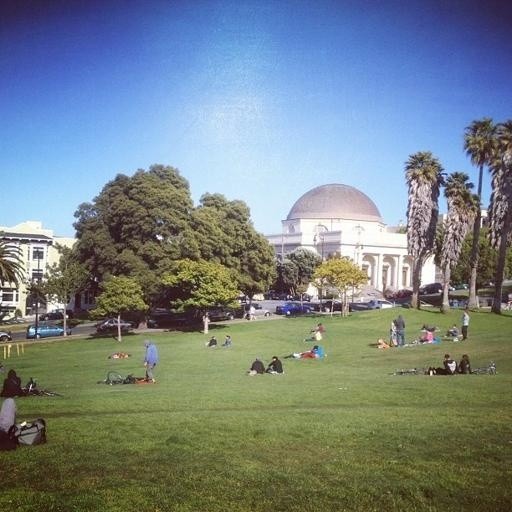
[388,289,413,298]
[317,300,353,313]
[236,303,271,320]
[276,302,311,316]
[39,309,72,321]
[96,318,132,332]
[368,298,394,310]
[0,331,13,342]
[449,281,469,291]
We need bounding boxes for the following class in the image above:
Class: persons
[249,306,256,320]
[456,354,472,374]
[141,340,159,384]
[390,310,469,347]
[301,322,326,360]
[221,335,233,352]
[266,356,284,373]
[428,353,457,375]
[0,369,27,397]
[251,357,265,374]
[205,336,218,349]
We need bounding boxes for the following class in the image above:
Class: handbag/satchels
[8,418,48,446]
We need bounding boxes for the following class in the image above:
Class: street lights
[312,234,326,313]
[32,246,44,340]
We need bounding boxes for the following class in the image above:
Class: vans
[26,324,73,340]
[419,283,442,295]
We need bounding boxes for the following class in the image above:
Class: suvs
[196,305,235,324]
